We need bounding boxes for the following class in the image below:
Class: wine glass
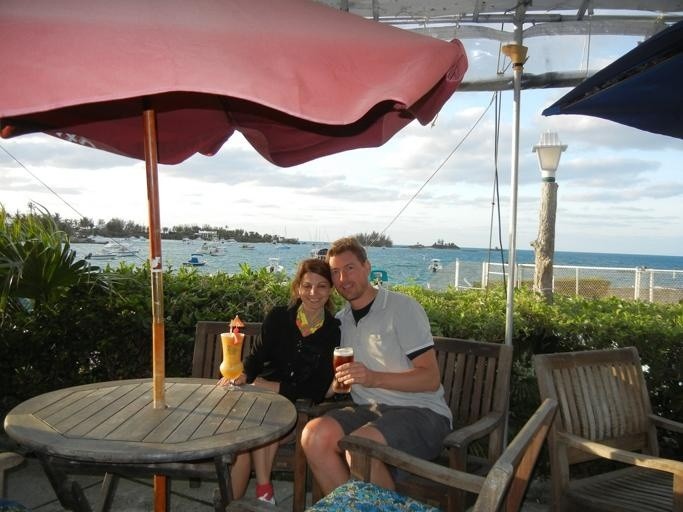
[220,332,244,391]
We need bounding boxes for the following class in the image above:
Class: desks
[4,376,295,512]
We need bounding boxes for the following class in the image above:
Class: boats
[241,243,254,249]
[183,239,236,267]
[310,248,328,263]
[429,258,442,271]
[267,240,290,272]
[87,242,138,260]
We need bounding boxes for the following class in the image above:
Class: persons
[217,258,340,507]
[297,236,453,500]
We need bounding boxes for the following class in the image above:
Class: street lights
[533,128,569,301]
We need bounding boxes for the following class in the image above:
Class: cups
[331,346,354,395]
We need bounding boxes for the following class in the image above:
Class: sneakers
[258,493,275,505]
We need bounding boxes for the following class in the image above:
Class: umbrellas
[0,0,468,511]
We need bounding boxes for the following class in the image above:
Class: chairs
[189,321,294,489]
[306,398,559,512]
[394,336,516,512]
[532,345,682,512]
